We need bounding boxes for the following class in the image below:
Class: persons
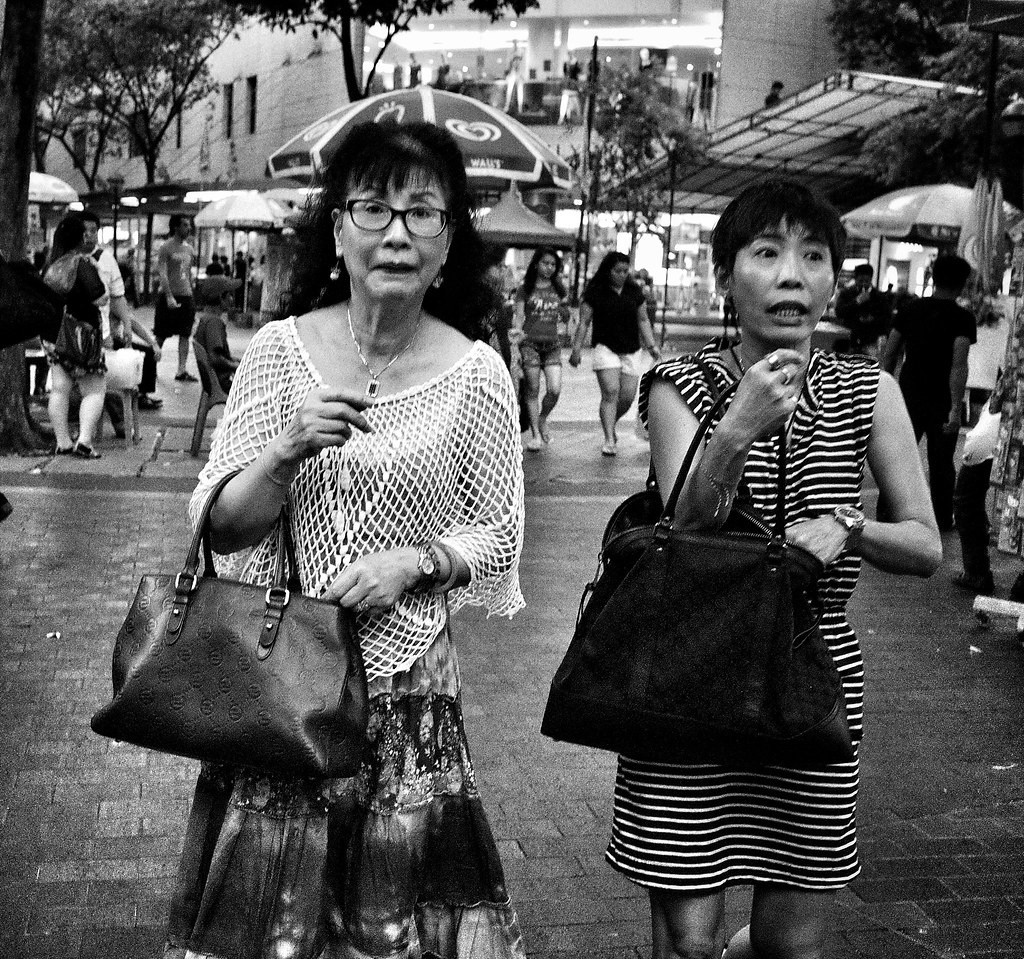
[723,299,739,335]
[504,39,527,115]
[156,121,528,959]
[508,247,662,454]
[834,255,1024,605]
[603,182,943,959]
[765,81,784,106]
[393,51,450,89]
[32,212,265,459]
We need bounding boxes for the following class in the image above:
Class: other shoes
[54,446,72,456]
[538,417,551,443]
[526,436,540,452]
[0,491,13,524]
[72,443,101,459]
[602,444,618,456]
[953,570,993,591]
[175,372,198,382]
[139,393,162,410]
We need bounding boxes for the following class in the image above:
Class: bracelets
[699,463,741,517]
[405,541,458,595]
[260,451,296,485]
[646,345,655,349]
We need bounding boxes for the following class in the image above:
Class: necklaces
[739,344,745,371]
[347,296,421,398]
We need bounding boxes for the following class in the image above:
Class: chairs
[190,338,230,458]
[39,339,143,449]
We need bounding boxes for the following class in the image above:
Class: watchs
[833,505,865,549]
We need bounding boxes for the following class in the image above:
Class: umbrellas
[28,171,79,203]
[193,191,291,311]
[842,182,1019,293]
[264,83,573,192]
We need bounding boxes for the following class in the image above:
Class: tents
[475,192,575,340]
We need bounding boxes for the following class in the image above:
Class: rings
[355,600,369,613]
[765,353,791,385]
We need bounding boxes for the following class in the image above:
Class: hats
[201,275,242,299]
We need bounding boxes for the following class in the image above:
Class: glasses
[340,199,452,238]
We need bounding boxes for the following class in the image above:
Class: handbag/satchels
[540,380,852,764]
[511,362,531,431]
[0,259,57,347]
[45,250,83,295]
[65,256,104,308]
[91,470,367,776]
